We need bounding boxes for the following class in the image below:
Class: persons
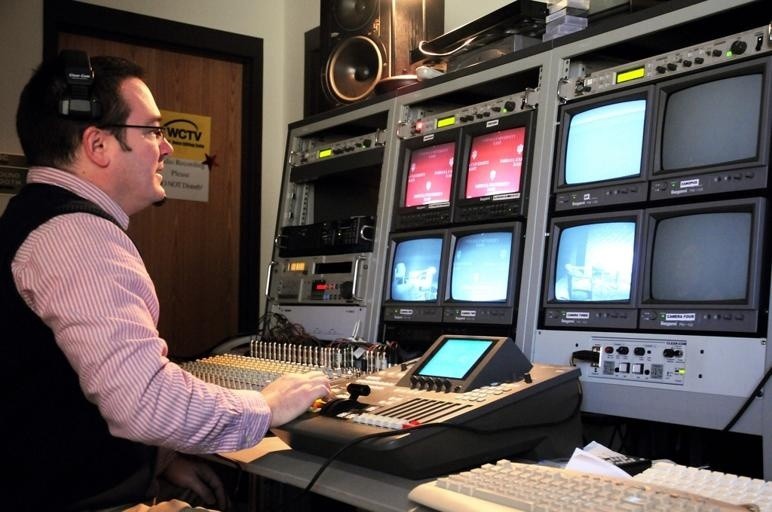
[0,49,330,511]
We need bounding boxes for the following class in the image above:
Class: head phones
[58,49,102,124]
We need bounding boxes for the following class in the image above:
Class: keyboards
[408,457,752,512]
[633,461,772,512]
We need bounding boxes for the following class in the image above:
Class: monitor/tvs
[381,229,450,323]
[637,196,767,334]
[441,222,525,326]
[390,127,463,233]
[553,84,656,213]
[543,209,646,330]
[646,55,772,202]
[453,110,538,227]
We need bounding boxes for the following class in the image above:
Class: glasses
[96,123,166,141]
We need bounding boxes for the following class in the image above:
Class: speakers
[319,0,444,116]
